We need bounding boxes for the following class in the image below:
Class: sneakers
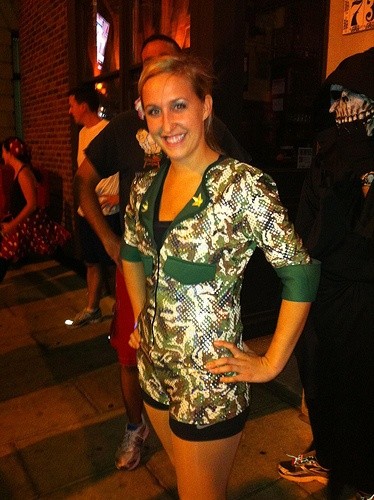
[112,422,149,470]
[277,453,330,484]
[67,307,103,330]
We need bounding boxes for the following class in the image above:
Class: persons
[64,84,122,329]
[272,44,374,500]
[73,34,252,472]
[121,52,323,500]
[1,136,73,287]
